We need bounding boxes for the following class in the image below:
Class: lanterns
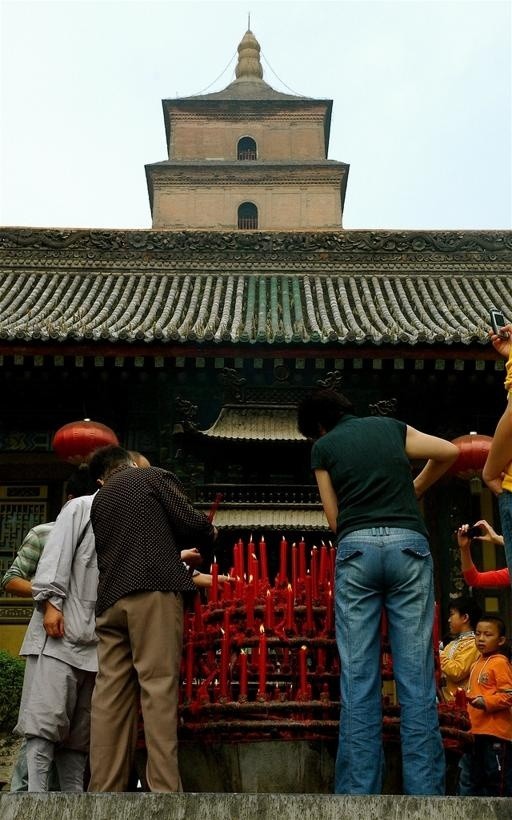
[51,418,120,471]
[446,431,493,495]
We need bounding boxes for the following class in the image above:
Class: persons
[482,324,512,585]
[438,597,480,701]
[0,487,96,792]
[457,519,511,588]
[457,614,512,796]
[85,442,213,793]
[296,386,462,795]
[12,448,153,792]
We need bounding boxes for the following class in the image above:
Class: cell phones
[491,311,508,338]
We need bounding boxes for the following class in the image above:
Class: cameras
[465,526,482,539]
